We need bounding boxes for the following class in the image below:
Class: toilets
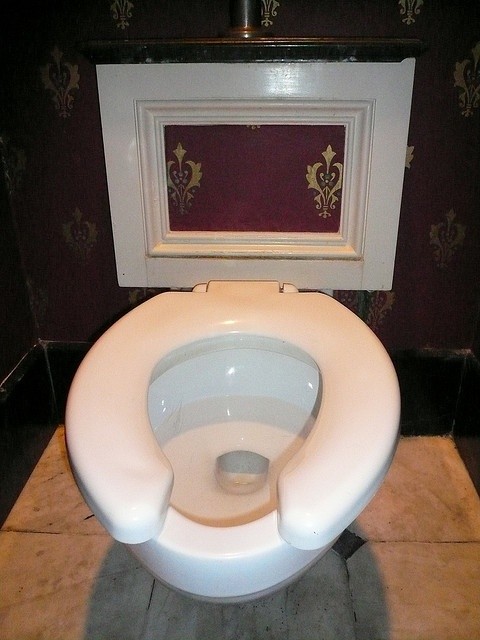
[67,281,402,604]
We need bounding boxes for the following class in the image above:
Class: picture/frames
[134,97,378,261]
